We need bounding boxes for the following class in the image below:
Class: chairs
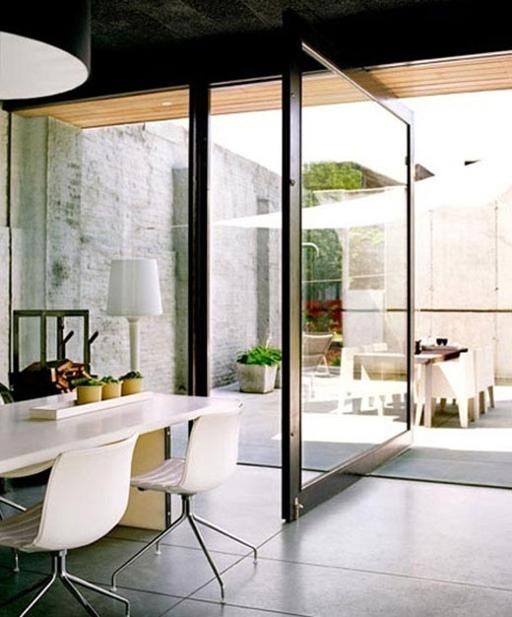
[110,404,258,608]
[340,341,498,427]
[0,433,149,617]
[301,331,336,398]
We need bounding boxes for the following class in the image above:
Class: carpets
[234,408,512,489]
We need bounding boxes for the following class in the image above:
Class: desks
[0,382,245,534]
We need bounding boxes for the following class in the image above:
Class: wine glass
[436,338,448,352]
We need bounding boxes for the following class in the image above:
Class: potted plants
[236,346,283,394]
[76,369,146,405]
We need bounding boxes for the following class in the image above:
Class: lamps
[104,257,165,375]
[0,0,92,102]
